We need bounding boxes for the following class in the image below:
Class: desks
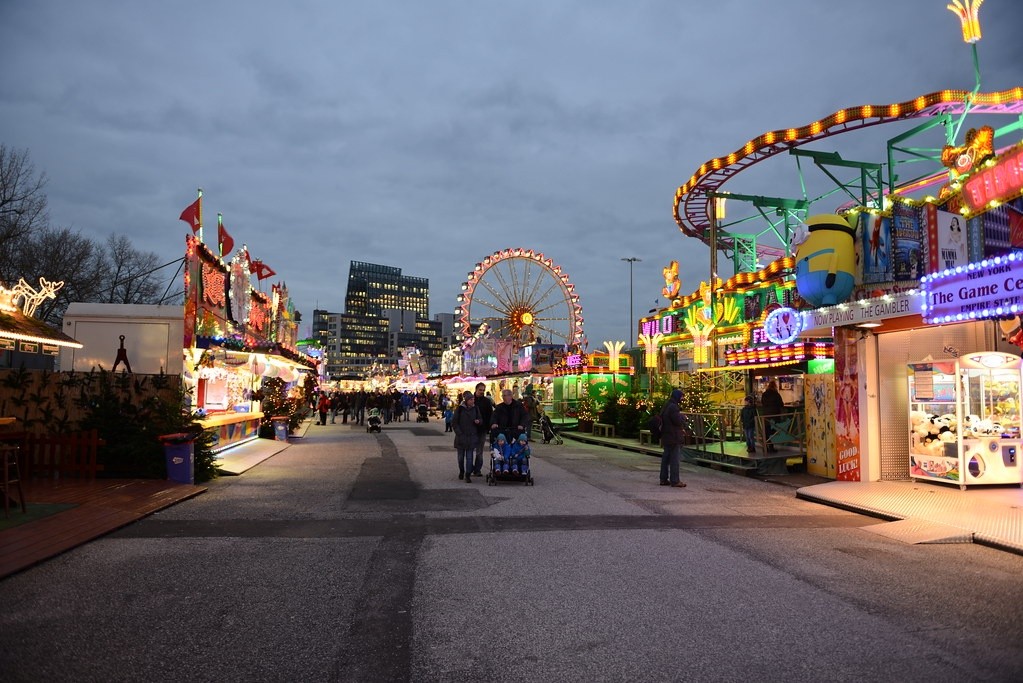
[0,427,31,480]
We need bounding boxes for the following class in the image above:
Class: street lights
[620,256,644,348]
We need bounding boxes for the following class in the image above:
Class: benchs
[640,430,663,447]
[593,423,615,437]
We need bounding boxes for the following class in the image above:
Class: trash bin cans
[158,434,200,485]
[271,416,291,443]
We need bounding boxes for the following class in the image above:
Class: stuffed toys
[910,412,1005,457]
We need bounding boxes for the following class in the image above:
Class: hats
[497,434,506,442]
[518,433,527,441]
[463,390,473,401]
[745,395,753,405]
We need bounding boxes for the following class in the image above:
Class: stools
[0,445,27,520]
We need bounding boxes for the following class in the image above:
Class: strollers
[487,426,534,485]
[429,408,440,419]
[539,415,564,445]
[366,415,382,433]
[416,403,430,423]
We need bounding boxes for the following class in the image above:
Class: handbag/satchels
[649,414,662,436]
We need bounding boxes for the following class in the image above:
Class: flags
[179,196,200,236]
[218,223,234,257]
[245,251,280,292]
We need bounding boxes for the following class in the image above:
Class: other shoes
[496,469,501,476]
[465,477,471,483]
[459,470,463,479]
[445,429,452,432]
[660,480,671,485]
[331,421,336,424]
[746,446,754,452]
[321,423,325,425]
[528,438,535,442]
[475,470,483,476]
[522,472,528,476]
[503,470,509,475]
[513,469,519,475]
[672,480,687,487]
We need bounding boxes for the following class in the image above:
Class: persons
[492,434,511,474]
[947,217,964,252]
[659,390,687,487]
[839,366,855,436]
[511,434,530,475]
[490,390,529,430]
[740,396,756,453]
[868,216,885,265]
[522,390,541,442]
[907,247,921,279]
[311,390,463,433]
[466,383,493,477]
[761,381,784,452]
[451,391,483,483]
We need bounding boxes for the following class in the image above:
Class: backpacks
[326,399,331,408]
